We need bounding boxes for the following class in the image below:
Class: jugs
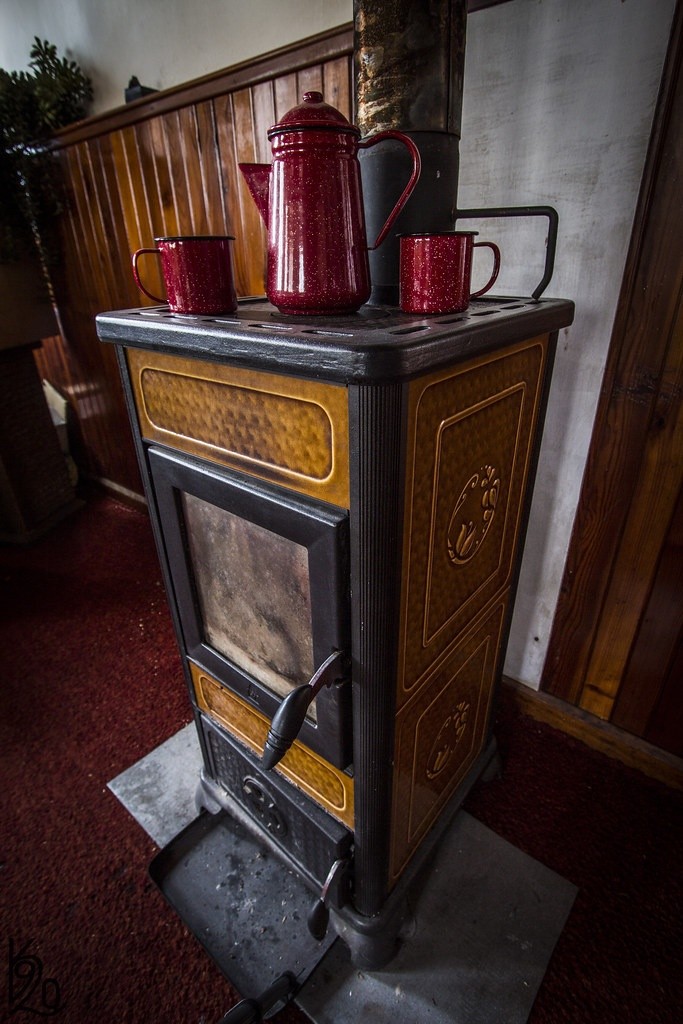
[238,91,421,316]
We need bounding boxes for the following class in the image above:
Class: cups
[132,236,237,318]
[395,232,501,315]
